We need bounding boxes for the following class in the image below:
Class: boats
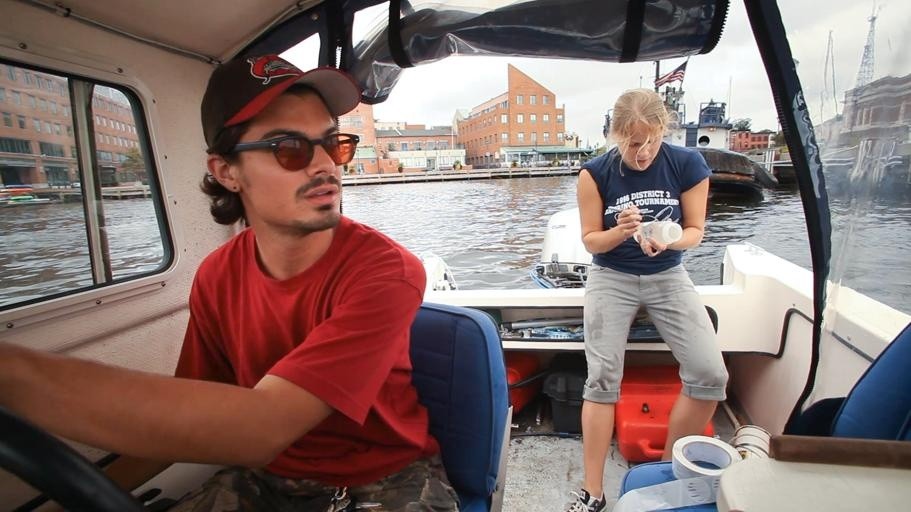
[602,99,779,199]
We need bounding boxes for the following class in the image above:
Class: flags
[653,60,686,89]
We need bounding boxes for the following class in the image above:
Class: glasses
[225,131,360,174]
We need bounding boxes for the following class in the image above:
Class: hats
[201,48,363,155]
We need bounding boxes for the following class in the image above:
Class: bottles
[633,221,683,246]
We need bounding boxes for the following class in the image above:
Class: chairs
[621,321,911,512]
[410,301,516,511]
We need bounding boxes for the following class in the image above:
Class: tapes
[612,435,744,510]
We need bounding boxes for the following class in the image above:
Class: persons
[566,87,730,512]
[0,55,460,512]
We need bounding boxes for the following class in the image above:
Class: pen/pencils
[609,206,645,217]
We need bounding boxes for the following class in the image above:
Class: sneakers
[563,486,609,512]
